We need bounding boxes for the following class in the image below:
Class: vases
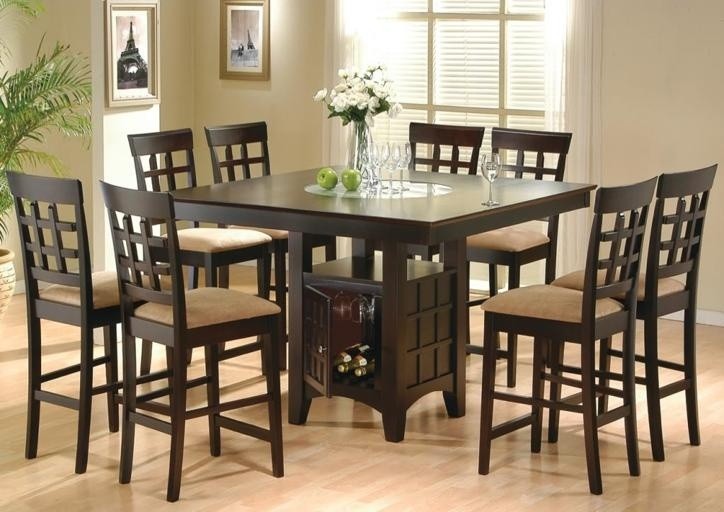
[345,119,377,189]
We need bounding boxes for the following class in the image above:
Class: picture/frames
[219,0,270,81]
[104,0,162,108]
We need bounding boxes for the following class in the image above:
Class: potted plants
[0,0,93,321]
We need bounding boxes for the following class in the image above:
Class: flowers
[313,63,402,173]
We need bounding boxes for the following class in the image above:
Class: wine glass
[480,153,502,206]
[332,288,376,326]
[357,141,412,194]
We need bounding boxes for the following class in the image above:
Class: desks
[163,166,597,445]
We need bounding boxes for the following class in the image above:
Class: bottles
[332,341,376,380]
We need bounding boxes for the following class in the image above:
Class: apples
[341,169,362,192]
[316,167,337,191]
